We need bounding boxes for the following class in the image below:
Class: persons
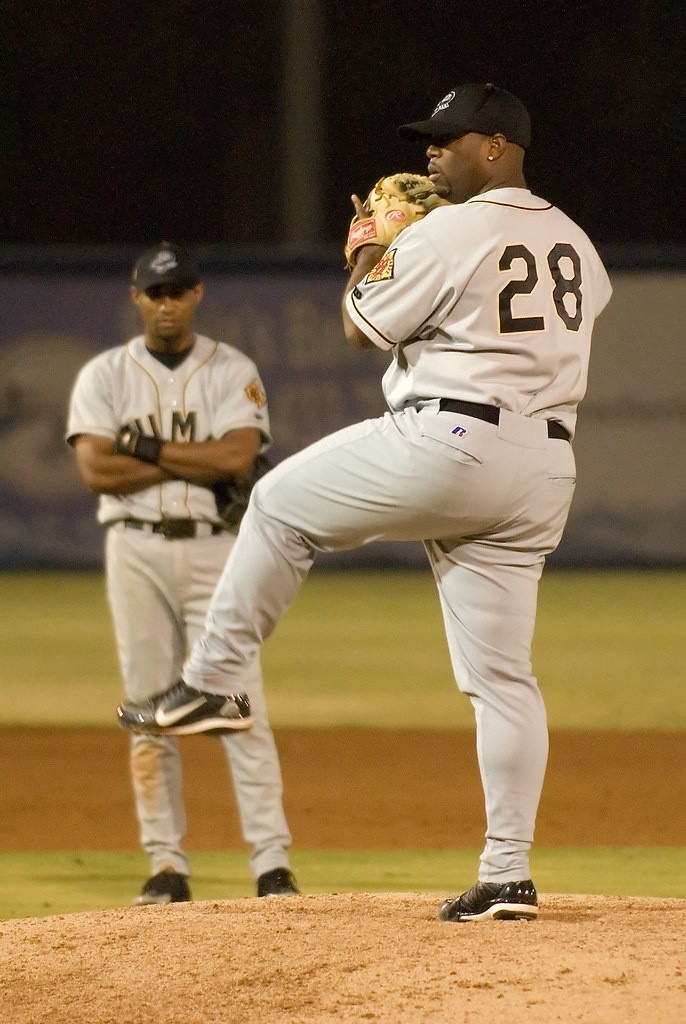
[66,237,302,905]
[117,78,613,923]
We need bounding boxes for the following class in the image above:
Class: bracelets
[134,436,164,466]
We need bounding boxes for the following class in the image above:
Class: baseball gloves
[345,171,457,270]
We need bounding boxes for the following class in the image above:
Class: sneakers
[117,680,253,736]
[436,878,539,921]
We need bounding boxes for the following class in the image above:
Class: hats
[130,242,199,290]
[398,82,532,149]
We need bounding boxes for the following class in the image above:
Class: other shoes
[257,868,301,896]
[135,873,192,903]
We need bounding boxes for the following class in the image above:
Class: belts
[123,518,221,538]
[440,400,571,443]
